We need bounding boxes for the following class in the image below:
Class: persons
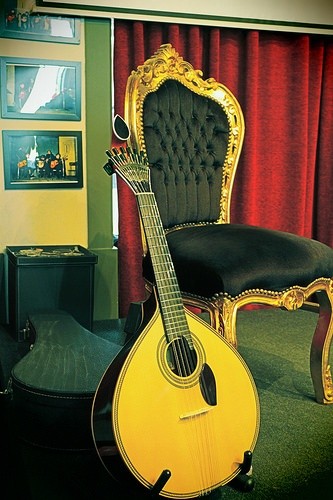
[7,10,44,32]
[18,83,25,101]
[17,146,62,179]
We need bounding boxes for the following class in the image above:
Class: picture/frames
[0,56,81,121]
[2,130,83,190]
[0,1,80,43]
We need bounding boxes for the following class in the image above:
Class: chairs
[122,43,333,405]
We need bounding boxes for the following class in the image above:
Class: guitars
[92,147,261,500]
[17,160,26,168]
[37,157,52,169]
[50,159,62,168]
